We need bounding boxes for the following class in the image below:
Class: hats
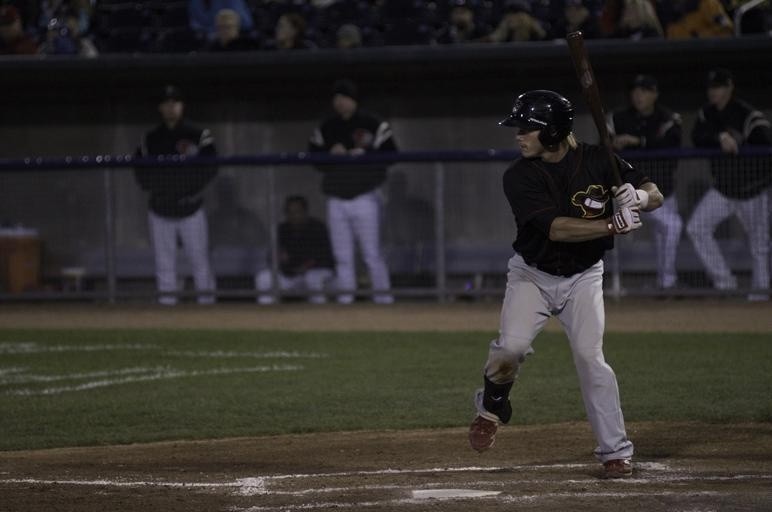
[331,77,360,102]
[706,68,732,87]
[626,72,657,90]
[155,84,185,101]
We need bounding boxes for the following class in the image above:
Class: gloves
[611,183,648,209]
[607,207,643,234]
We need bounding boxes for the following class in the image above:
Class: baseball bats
[565,30,625,186]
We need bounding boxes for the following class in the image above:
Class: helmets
[496,90,575,152]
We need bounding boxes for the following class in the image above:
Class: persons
[253,193,336,303]
[2,1,771,53]
[682,68,771,304]
[600,71,684,290]
[130,84,221,307]
[466,86,667,479]
[306,81,402,305]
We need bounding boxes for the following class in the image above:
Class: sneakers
[468,415,498,451]
[604,457,632,479]
